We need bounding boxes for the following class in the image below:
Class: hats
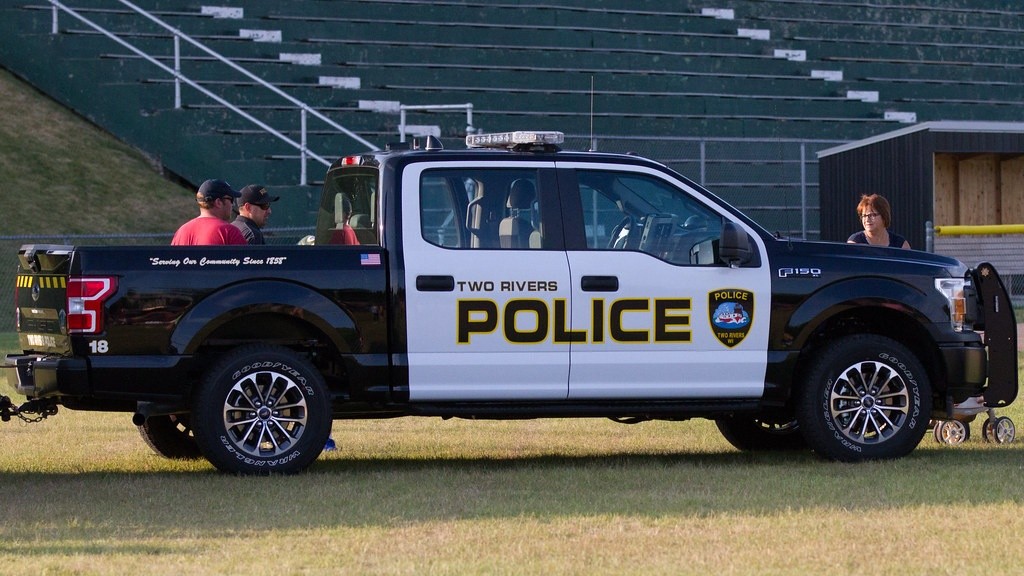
[236,184,280,206]
[195,179,242,202]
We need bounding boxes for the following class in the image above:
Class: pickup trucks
[1,132,993,477]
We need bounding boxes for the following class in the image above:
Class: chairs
[332,190,379,230]
[499,180,544,249]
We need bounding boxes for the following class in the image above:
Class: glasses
[861,213,881,219]
[223,197,234,203]
[250,202,270,210]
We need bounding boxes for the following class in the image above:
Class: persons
[230,184,272,245]
[846,192,910,249]
[169,179,247,245]
[322,196,361,245]
